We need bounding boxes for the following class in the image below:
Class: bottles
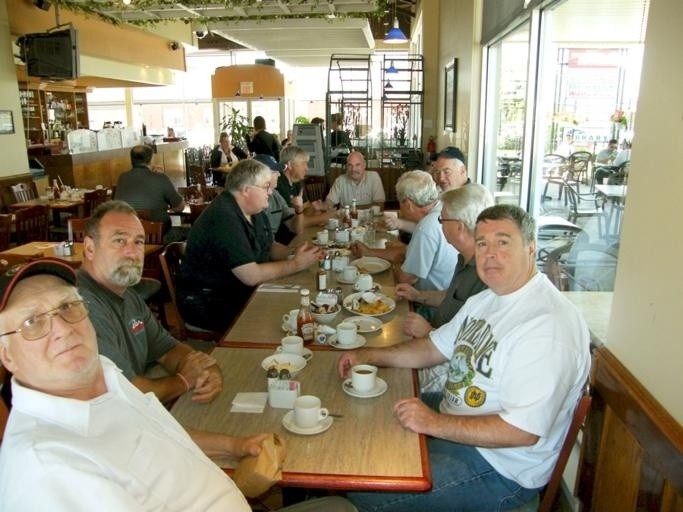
[265,366,291,392]
[40,179,103,201]
[19,90,36,116]
[102,121,124,130]
[311,198,392,292]
[297,289,315,344]
[187,184,204,206]
[53,240,74,257]
[47,97,73,141]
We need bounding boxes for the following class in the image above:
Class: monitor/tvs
[16,29,80,82]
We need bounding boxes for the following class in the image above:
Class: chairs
[497,151,630,291]
[539,383,592,512]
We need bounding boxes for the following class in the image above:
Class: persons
[1,257,358,511]
[326,151,385,209]
[179,115,335,334]
[114,145,190,246]
[330,116,352,149]
[593,139,631,185]
[74,199,222,412]
[382,146,494,393]
[339,205,592,511]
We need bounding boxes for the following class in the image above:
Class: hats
[429,147,465,162]
[0,256,78,313]
[252,154,287,173]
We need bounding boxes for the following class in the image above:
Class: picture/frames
[0,110,15,135]
[444,58,457,133]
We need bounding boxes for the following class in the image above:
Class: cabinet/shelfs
[16,64,90,155]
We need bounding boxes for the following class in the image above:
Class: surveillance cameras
[191,24,208,39]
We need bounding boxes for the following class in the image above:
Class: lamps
[382,60,399,101]
[384,0,408,43]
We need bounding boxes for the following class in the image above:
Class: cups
[281,336,304,354]
[351,365,378,393]
[293,395,329,426]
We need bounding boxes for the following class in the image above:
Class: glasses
[438,214,460,225]
[247,183,271,193]
[0,300,90,341]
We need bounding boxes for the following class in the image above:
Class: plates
[342,377,388,398]
[281,292,396,349]
[261,348,313,374]
[283,409,333,434]
